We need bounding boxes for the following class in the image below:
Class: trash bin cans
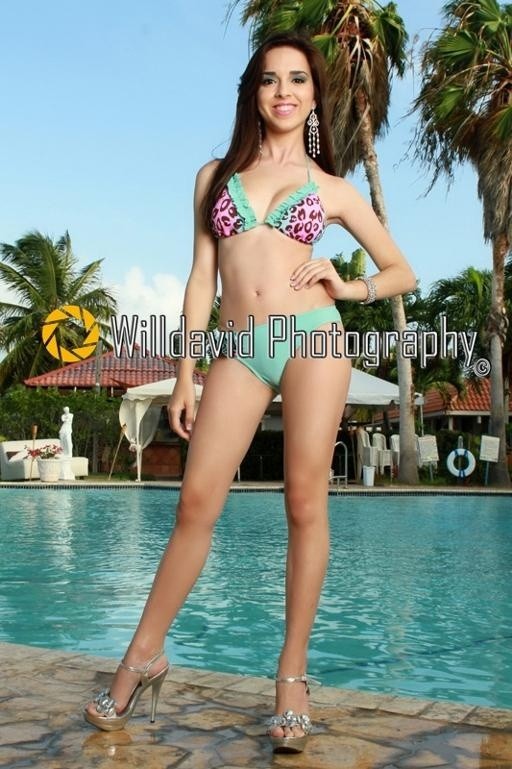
[363,465,375,487]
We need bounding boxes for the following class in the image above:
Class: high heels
[83,649,170,730]
[266,676,311,751]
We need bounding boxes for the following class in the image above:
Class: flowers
[23,442,65,460]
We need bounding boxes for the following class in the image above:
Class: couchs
[2,437,92,479]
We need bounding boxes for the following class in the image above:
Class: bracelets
[355,275,377,305]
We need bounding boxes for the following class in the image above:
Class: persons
[59,406,74,457]
[83,33,415,754]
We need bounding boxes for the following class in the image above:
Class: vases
[37,459,64,483]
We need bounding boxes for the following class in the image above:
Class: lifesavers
[447,449,477,478]
